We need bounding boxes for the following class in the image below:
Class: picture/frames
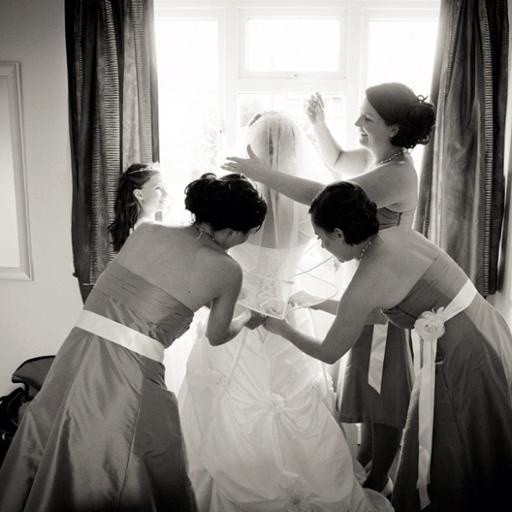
[0,58,35,286]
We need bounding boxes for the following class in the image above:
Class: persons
[0,162,268,512]
[108,163,169,254]
[263,179,511,511]
[177,109,394,511]
[222,82,436,494]
